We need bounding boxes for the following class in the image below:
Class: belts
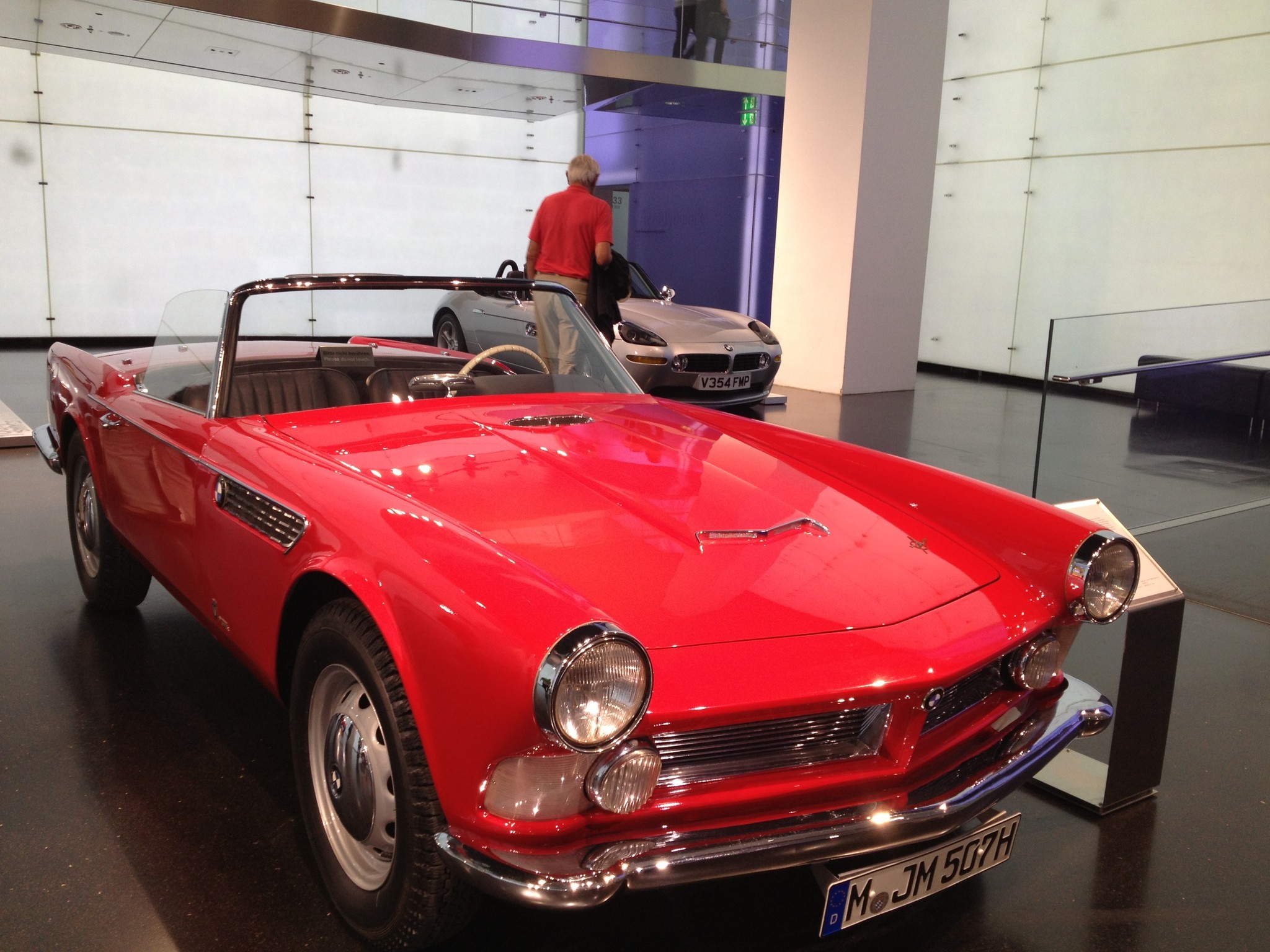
[538,272,588,283]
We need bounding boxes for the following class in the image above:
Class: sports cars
[430,250,783,413]
[28,257,1144,952]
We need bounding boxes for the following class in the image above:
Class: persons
[673,0,731,64]
[526,154,614,375]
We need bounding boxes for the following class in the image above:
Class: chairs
[506,269,523,279]
[366,365,496,402]
[184,366,363,416]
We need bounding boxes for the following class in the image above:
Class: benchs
[1133,352,1270,432]
